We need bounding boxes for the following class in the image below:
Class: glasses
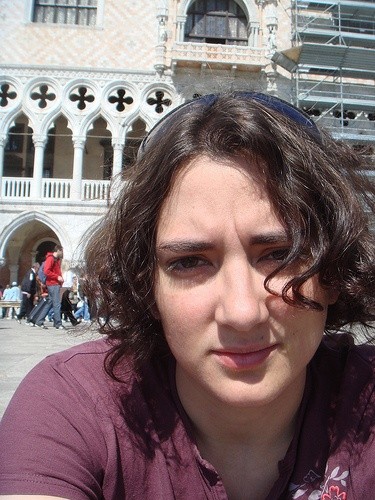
[131,89,323,171]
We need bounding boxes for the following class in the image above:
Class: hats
[12,281,17,286]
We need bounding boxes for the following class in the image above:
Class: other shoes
[76,321,81,325]
[6,317,12,320]
[14,315,66,330]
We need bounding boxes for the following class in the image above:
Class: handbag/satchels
[76,300,84,308]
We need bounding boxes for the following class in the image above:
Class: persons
[0,282,23,320]
[0,90,375,500]
[60,276,97,327]
[13,245,65,331]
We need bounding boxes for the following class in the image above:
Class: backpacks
[37,255,56,285]
